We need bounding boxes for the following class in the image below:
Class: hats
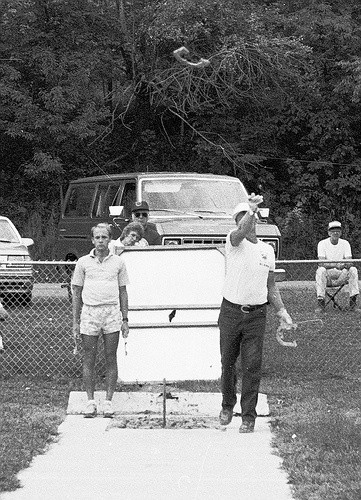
[328,221,342,231]
[233,203,258,219]
[131,201,149,212]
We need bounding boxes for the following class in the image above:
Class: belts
[222,297,268,313]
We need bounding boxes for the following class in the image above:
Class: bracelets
[122,317,129,322]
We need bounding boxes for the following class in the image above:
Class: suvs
[54,171,283,304]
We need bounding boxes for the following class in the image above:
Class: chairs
[325,281,349,312]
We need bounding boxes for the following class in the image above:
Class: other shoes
[83,401,98,418]
[102,405,114,418]
[239,421,255,433]
[219,406,233,425]
[349,301,361,313]
[314,302,325,313]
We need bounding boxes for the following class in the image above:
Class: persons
[71,223,129,418]
[315,221,360,313]
[108,201,162,256]
[218,193,294,433]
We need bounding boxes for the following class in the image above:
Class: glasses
[134,212,148,217]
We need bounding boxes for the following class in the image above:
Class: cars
[0,216,35,308]
